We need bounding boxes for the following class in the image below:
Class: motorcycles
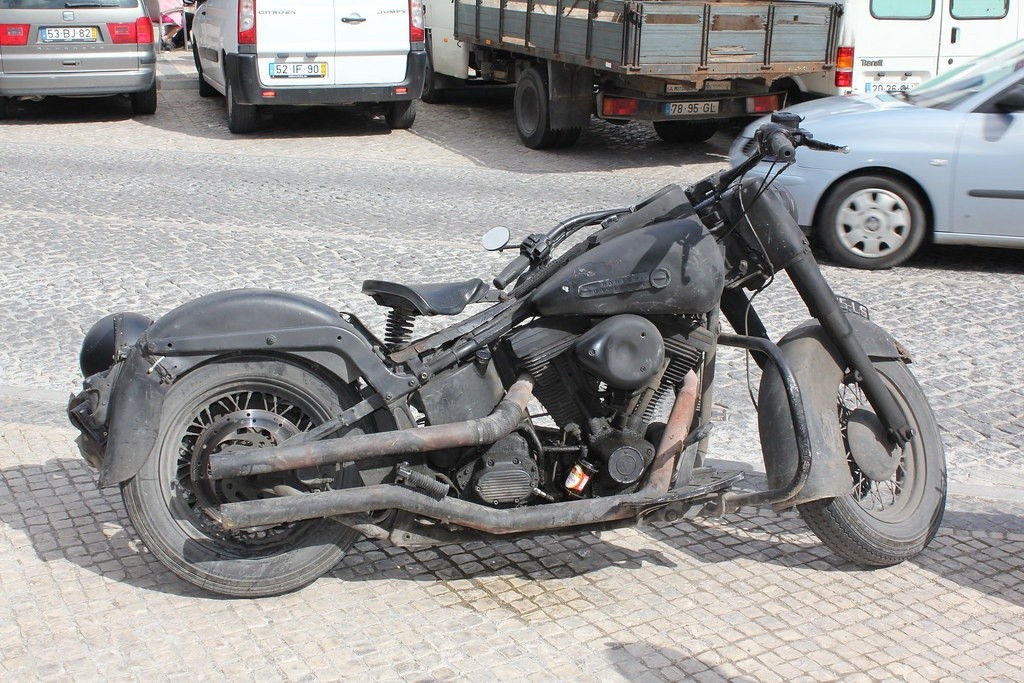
[67,112,949,603]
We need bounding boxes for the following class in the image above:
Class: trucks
[420,0,843,156]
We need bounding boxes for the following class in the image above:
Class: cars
[1,1,158,117]
[728,40,1024,273]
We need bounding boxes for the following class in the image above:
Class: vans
[779,0,1023,114]
[183,0,420,136]
